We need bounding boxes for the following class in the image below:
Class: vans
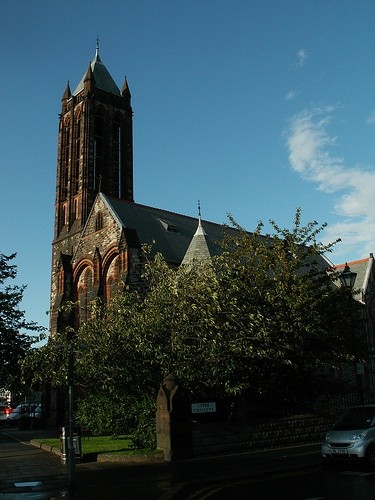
[7,404,38,420]
[321,405,375,472]
[28,404,41,418]
